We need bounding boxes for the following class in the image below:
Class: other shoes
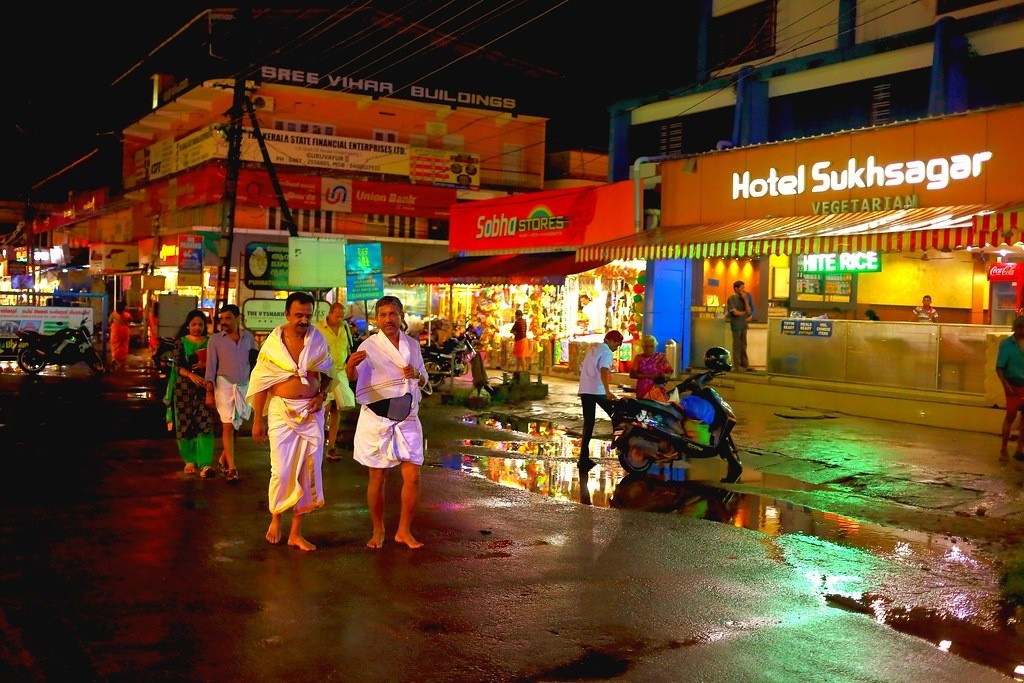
[326,448,343,461]
[731,366,746,372]
[226,468,239,483]
[1013,451,1024,460]
[999,449,1010,461]
[184,465,197,474]
[577,459,595,468]
[217,462,229,476]
[745,367,755,372]
[200,466,217,478]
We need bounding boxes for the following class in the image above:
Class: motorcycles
[150,335,175,375]
[13,309,106,374]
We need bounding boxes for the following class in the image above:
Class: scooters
[422,324,481,388]
[607,367,745,477]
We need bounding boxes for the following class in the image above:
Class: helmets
[704,347,733,372]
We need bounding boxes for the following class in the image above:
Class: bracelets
[206,390,214,392]
[187,371,191,377]
[418,371,423,378]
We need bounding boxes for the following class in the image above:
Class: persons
[722,281,755,372]
[864,309,880,321]
[245,292,335,552]
[511,295,624,468]
[314,303,355,463]
[996,315,1024,462]
[345,297,429,548]
[108,301,258,482]
[630,334,674,403]
[909,295,939,323]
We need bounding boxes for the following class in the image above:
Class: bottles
[806,280,810,293]
[799,280,802,292]
[809,280,814,293]
[837,282,841,294]
[802,281,806,292]
[797,267,805,279]
[815,283,819,294]
[797,279,800,292]
[845,282,849,294]
[841,282,845,294]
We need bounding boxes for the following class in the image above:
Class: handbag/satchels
[682,417,711,446]
[680,395,715,424]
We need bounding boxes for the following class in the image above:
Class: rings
[196,380,199,383]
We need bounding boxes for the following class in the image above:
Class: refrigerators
[987,262,1024,325]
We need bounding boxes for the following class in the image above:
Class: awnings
[575,201,1024,263]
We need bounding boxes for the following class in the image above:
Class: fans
[902,251,954,261]
